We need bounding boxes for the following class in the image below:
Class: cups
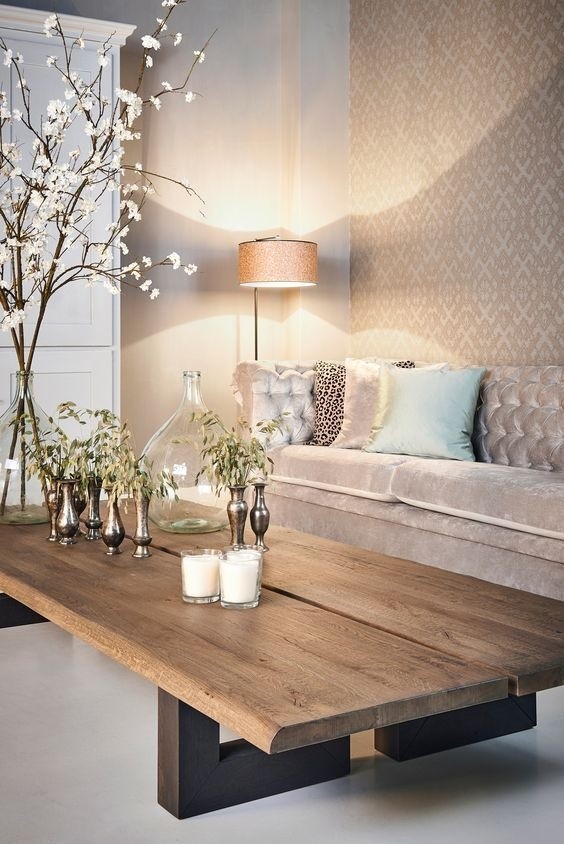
[220,554,264,609]
[224,545,265,556]
[181,549,222,603]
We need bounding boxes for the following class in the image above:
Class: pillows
[307,360,487,462]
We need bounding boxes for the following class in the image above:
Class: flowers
[0,0,219,518]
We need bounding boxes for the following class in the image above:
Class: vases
[0,370,71,525]
[139,371,247,535]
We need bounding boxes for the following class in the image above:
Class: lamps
[237,235,319,360]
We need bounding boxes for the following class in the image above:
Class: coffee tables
[0,498,563,820]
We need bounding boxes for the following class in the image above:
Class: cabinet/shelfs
[0,6,136,632]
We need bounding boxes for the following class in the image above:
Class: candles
[181,550,261,604]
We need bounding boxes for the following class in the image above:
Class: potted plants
[173,409,297,554]
[6,399,180,560]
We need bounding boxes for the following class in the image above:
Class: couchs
[230,359,564,604]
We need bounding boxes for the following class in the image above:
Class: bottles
[0,370,73,525]
[138,372,238,533]
